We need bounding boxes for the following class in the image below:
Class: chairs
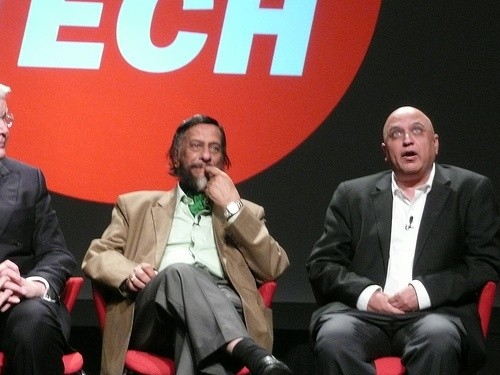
[373,281,495,375]
[91,270,278,375]
[0,275,86,375]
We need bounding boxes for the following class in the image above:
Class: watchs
[224,200,243,221]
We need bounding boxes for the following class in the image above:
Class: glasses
[0,113,13,128]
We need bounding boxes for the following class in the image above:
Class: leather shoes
[257,356,289,375]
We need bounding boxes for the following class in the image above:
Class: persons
[307,106,500,375]
[0,84,79,375]
[81,115,295,375]
